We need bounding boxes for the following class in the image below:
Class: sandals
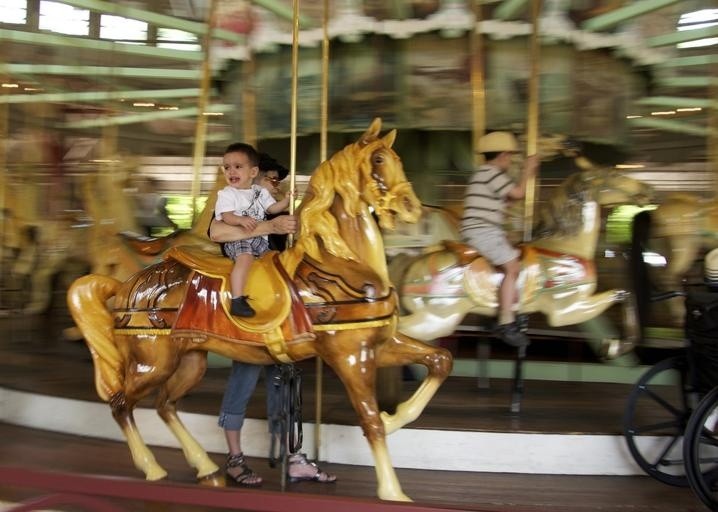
[287,453,337,485]
[226,452,262,486]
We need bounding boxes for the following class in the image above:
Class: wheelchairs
[620,276,718,512]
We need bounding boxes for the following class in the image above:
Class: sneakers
[494,320,529,345]
[229,295,255,316]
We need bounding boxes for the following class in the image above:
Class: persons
[685,248,718,433]
[208,143,337,486]
[461,132,540,348]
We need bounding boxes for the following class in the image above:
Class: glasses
[266,175,280,186]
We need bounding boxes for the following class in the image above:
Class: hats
[703,248,718,283]
[259,154,290,180]
[475,128,522,154]
